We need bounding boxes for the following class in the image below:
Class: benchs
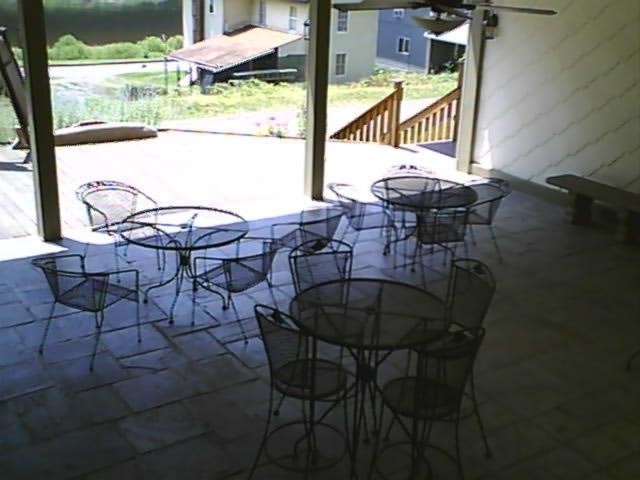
[545,173,640,227]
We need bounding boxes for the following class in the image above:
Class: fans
[332,1,558,37]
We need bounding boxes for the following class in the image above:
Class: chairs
[379,164,443,240]
[271,202,366,303]
[445,176,512,262]
[368,325,490,479]
[191,238,278,344]
[415,256,495,442]
[329,183,398,270]
[273,237,354,419]
[408,209,475,293]
[248,302,363,480]
[30,253,142,372]
[76,178,161,285]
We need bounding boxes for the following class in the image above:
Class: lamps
[410,14,467,38]
